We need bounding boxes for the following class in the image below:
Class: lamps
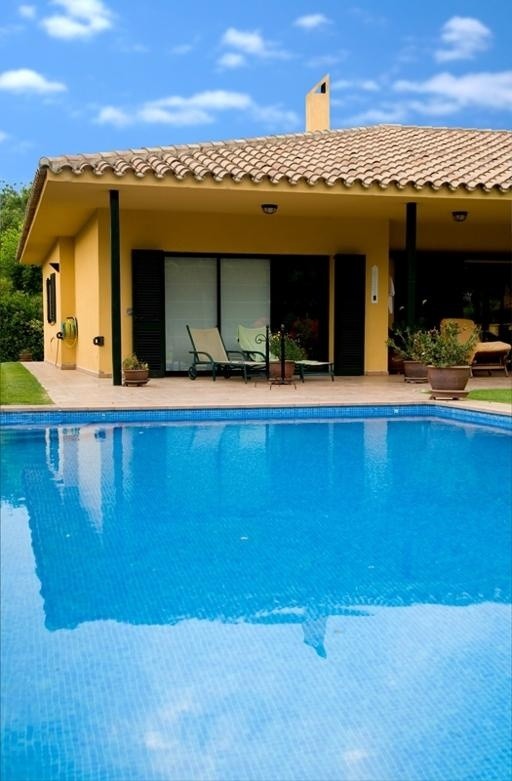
[452,211,468,221]
[261,204,278,214]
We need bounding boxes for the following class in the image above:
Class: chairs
[237,324,335,382]
[440,318,512,379]
[186,325,266,385]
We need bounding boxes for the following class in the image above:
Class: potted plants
[123,352,149,380]
[385,326,427,377]
[269,333,306,377]
[416,321,483,390]
